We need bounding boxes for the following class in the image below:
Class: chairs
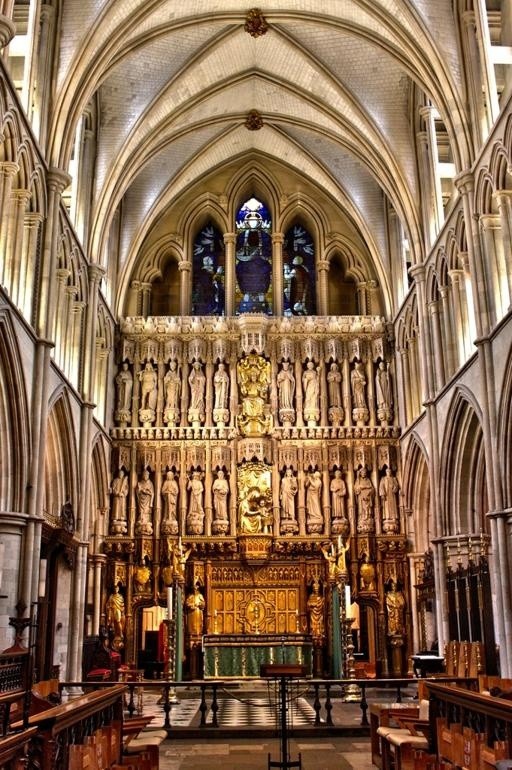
[432,639,485,692]
[376,699,429,770]
[123,715,168,770]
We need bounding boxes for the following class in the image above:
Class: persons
[279,468,400,521]
[198,256,224,315]
[187,585,205,637]
[114,470,229,522]
[283,256,311,314]
[277,360,394,415]
[238,490,273,535]
[114,358,230,413]
[240,370,268,421]
[106,586,126,638]
[307,584,325,637]
[384,583,405,635]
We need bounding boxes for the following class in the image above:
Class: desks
[369,702,419,752]
